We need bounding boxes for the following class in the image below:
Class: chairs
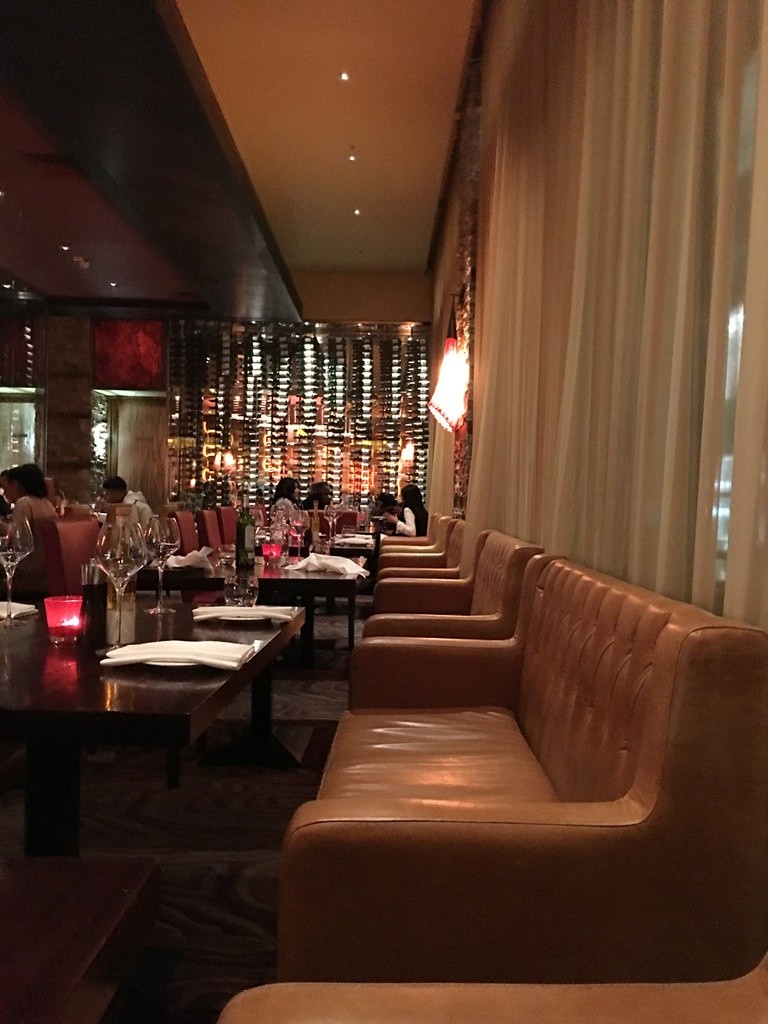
[308,512,324,519]
[247,505,269,526]
[168,510,201,556]
[217,507,238,546]
[310,518,330,542]
[336,512,359,533]
[41,514,100,598]
[64,504,90,515]
[106,503,132,525]
[197,510,221,550]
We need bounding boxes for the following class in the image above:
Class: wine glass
[288,510,311,565]
[256,527,270,565]
[0,516,34,626]
[323,504,342,545]
[144,516,181,615]
[94,515,149,655]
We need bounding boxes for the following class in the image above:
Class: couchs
[214,514,768,1024]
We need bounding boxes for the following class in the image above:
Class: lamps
[424,292,474,432]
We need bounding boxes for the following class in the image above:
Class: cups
[308,545,330,555]
[357,506,372,527]
[218,544,236,568]
[250,509,263,534]
[262,544,282,565]
[224,575,259,607]
[44,596,83,648]
[341,525,356,538]
[107,572,137,608]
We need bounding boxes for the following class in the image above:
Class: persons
[103,476,153,528]
[299,482,331,510]
[0,464,56,579]
[270,477,301,516]
[383,485,428,537]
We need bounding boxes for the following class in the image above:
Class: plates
[319,533,327,537]
[220,616,267,621]
[341,539,368,545]
[143,661,199,666]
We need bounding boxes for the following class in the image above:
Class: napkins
[0,601,40,619]
[336,534,372,539]
[98,640,256,669]
[166,545,215,572]
[335,537,374,544]
[286,551,371,581]
[191,606,300,624]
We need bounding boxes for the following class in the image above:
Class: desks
[0,606,305,858]
[256,532,377,614]
[81,556,368,672]
[0,858,183,1024]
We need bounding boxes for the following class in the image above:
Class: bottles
[235,494,255,569]
[270,508,290,566]
[80,558,107,602]
[165,331,429,510]
[310,500,319,542]
